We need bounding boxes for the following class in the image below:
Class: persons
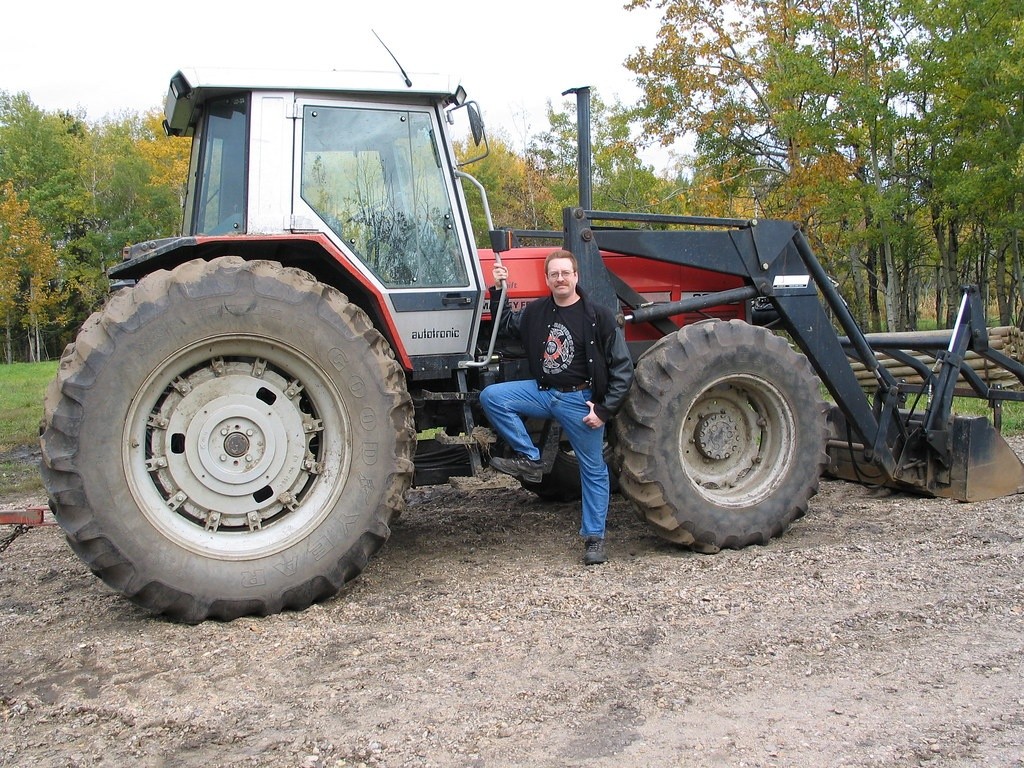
[480,251,635,565]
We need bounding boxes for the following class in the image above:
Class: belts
[553,382,590,392]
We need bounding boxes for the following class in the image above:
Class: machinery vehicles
[38,66,1023,624]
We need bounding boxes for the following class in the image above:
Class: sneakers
[584,536,609,565]
[488,452,547,483]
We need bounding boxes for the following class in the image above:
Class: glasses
[547,270,576,278]
